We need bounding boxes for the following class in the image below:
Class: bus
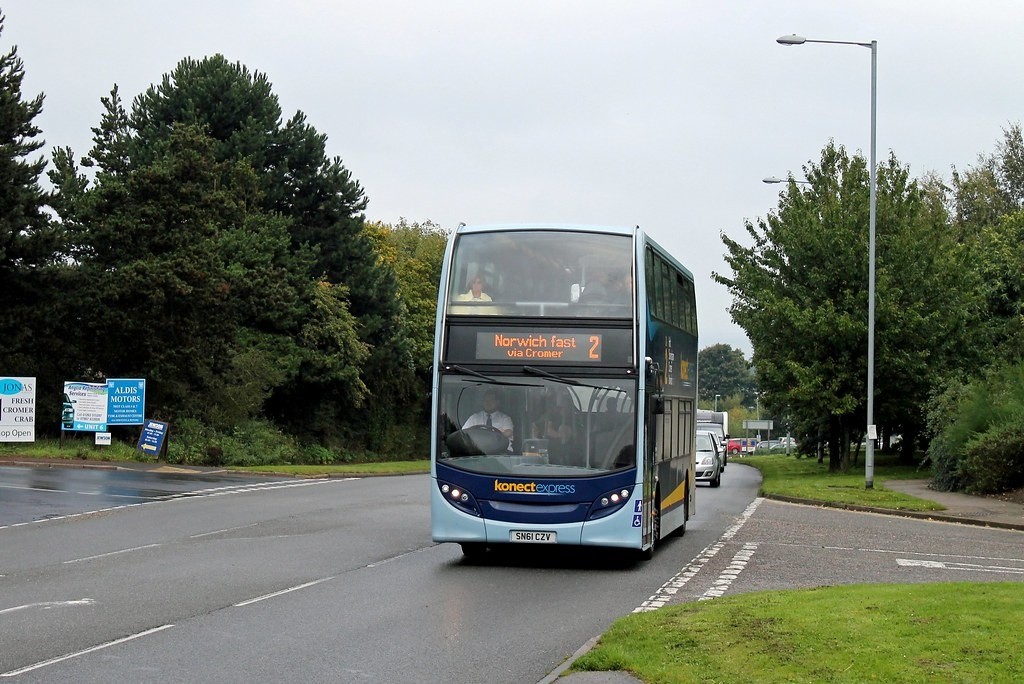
[430,222,700,554]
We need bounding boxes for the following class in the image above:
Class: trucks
[697,409,728,464]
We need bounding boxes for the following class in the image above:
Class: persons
[529,411,571,439]
[456,275,493,302]
[462,389,513,452]
[606,397,618,412]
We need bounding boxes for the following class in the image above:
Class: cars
[740,437,797,450]
[726,440,741,454]
[696,430,726,488]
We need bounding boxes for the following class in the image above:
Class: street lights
[779,35,877,493]
[749,392,760,434]
[715,394,721,412]
